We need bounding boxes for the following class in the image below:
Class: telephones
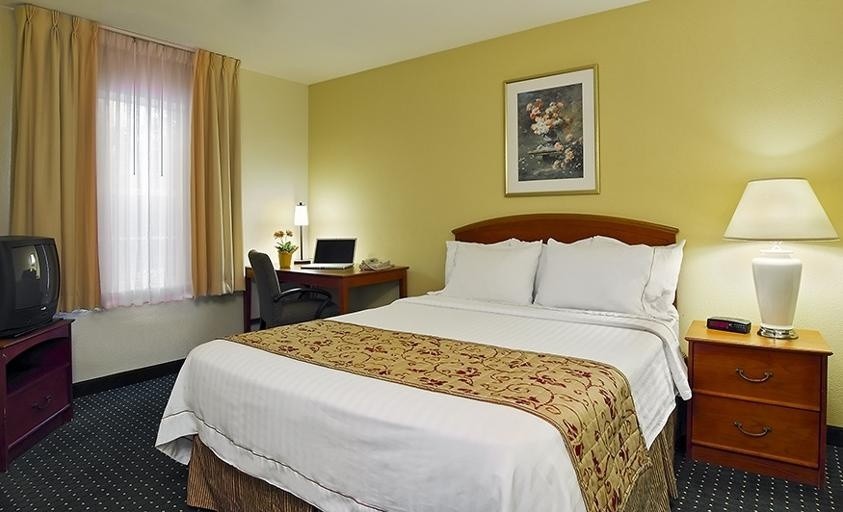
[359,258,392,270]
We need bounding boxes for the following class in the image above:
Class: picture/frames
[502,63,602,196]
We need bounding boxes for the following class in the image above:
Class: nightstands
[685,318,834,490]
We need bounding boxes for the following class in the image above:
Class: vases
[278,251,293,269]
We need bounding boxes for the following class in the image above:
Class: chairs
[249,249,340,330]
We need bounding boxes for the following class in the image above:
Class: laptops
[301,237,357,270]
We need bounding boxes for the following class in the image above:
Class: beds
[154,211,692,512]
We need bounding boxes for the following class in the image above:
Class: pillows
[534,240,654,317]
[594,232,686,320]
[448,239,542,305]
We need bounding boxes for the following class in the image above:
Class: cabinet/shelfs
[2,318,76,476]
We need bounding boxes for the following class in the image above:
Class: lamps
[723,178,840,341]
[293,202,312,264]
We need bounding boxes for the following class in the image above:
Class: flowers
[274,231,297,252]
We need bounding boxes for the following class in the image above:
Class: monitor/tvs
[0,235,62,338]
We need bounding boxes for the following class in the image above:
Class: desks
[244,264,410,332]
